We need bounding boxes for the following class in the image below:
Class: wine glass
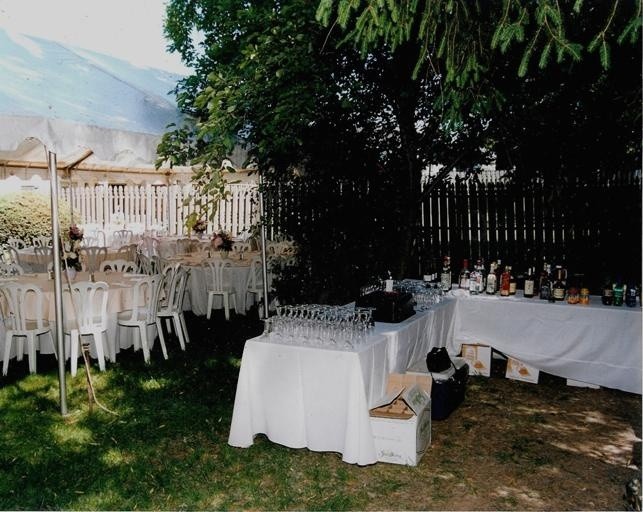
[260,304,377,350]
[358,279,444,312]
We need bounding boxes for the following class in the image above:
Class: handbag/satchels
[426,347,451,373]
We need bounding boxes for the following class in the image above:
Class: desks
[226,278,453,466]
[453,283,642,395]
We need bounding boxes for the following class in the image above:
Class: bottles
[423,255,637,307]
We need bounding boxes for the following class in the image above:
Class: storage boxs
[462,343,491,378]
[369,374,433,466]
[505,356,540,385]
[405,355,469,422]
